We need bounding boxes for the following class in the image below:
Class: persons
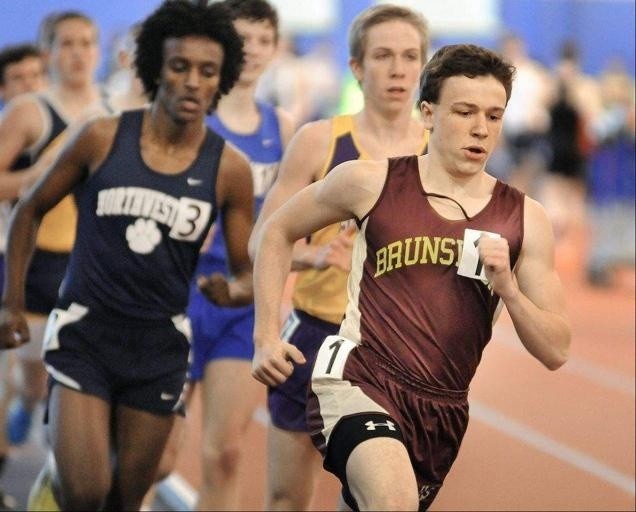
[249,6,429,511]
[250,45,573,511]
[264,18,635,287]
[136,0,292,512]
[98,24,160,117]
[1,45,56,320]
[1,0,254,512]
[1,13,116,459]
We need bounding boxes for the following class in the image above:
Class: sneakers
[6,397,31,445]
